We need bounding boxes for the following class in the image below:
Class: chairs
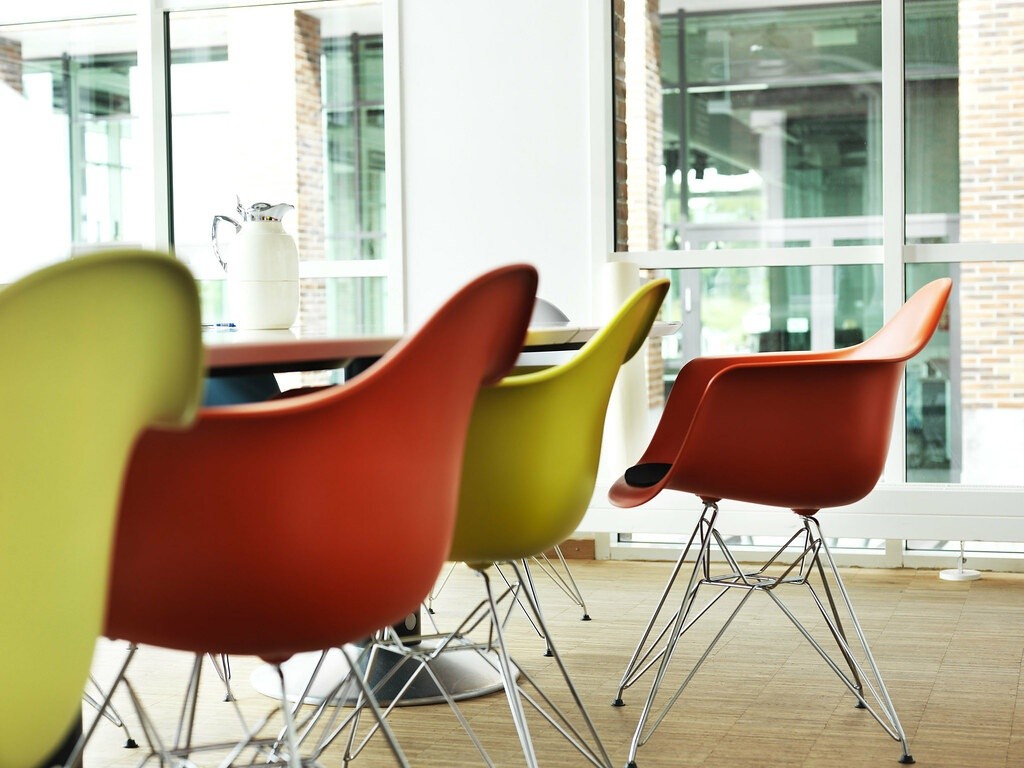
[71,262,540,767]
[3,246,201,767]
[263,277,669,767]
[606,276,958,768]
[429,297,596,657]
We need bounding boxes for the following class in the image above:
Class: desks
[190,325,604,706]
